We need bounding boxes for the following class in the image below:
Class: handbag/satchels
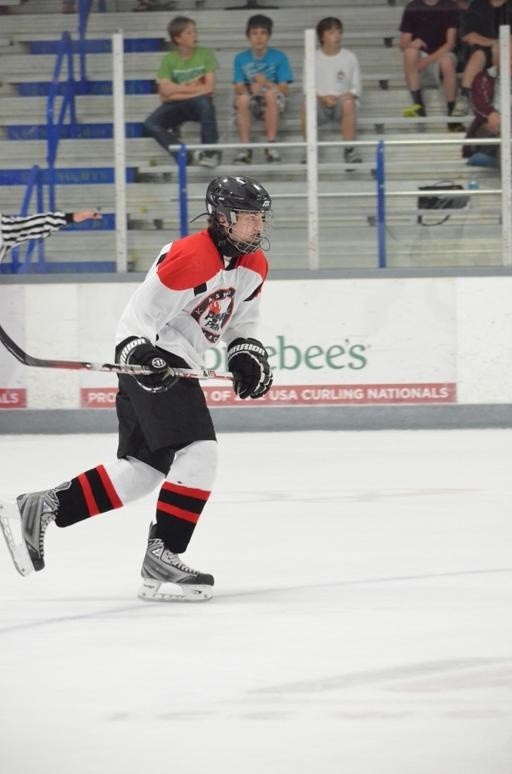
[417,179,467,226]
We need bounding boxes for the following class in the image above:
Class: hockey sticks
[0,328,243,380]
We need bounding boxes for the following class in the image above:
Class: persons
[459,34,511,192]
[13,171,277,588]
[138,12,222,169]
[396,0,467,133]
[448,0,512,119]
[229,13,296,163]
[300,15,368,173]
[0,204,107,265]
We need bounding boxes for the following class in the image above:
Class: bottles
[467,173,480,211]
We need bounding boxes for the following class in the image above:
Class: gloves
[114,337,178,395]
[225,338,274,397]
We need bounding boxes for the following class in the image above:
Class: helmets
[206,174,272,254]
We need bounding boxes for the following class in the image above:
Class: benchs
[1,0,512,274]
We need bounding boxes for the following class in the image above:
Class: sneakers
[185,151,200,166]
[265,143,283,164]
[341,148,362,172]
[402,103,427,122]
[447,118,467,134]
[197,150,222,167]
[234,147,252,164]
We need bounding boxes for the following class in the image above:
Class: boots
[141,522,215,588]
[15,481,71,572]
